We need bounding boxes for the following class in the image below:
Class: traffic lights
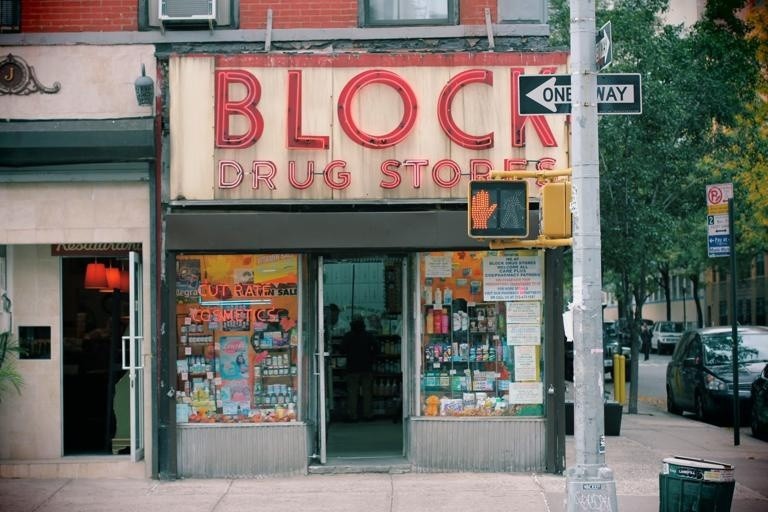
[467,180,572,239]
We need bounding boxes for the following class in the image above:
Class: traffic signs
[518,73,642,116]
[596,20,613,72]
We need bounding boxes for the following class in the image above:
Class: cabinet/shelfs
[422,301,498,397]
[178,309,295,413]
[332,336,401,421]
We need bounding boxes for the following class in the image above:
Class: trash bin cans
[659,456,736,512]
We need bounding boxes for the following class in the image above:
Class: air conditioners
[158,0,217,35]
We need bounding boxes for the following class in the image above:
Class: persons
[640,321,654,361]
[340,313,375,422]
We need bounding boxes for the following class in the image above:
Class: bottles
[181,322,212,343]
[434,287,442,304]
[427,305,450,333]
[470,346,496,360]
[254,356,296,375]
[261,391,295,405]
[187,355,206,372]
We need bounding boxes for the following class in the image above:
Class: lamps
[84,256,130,293]
[135,64,154,105]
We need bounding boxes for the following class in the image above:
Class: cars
[565,318,768,441]
